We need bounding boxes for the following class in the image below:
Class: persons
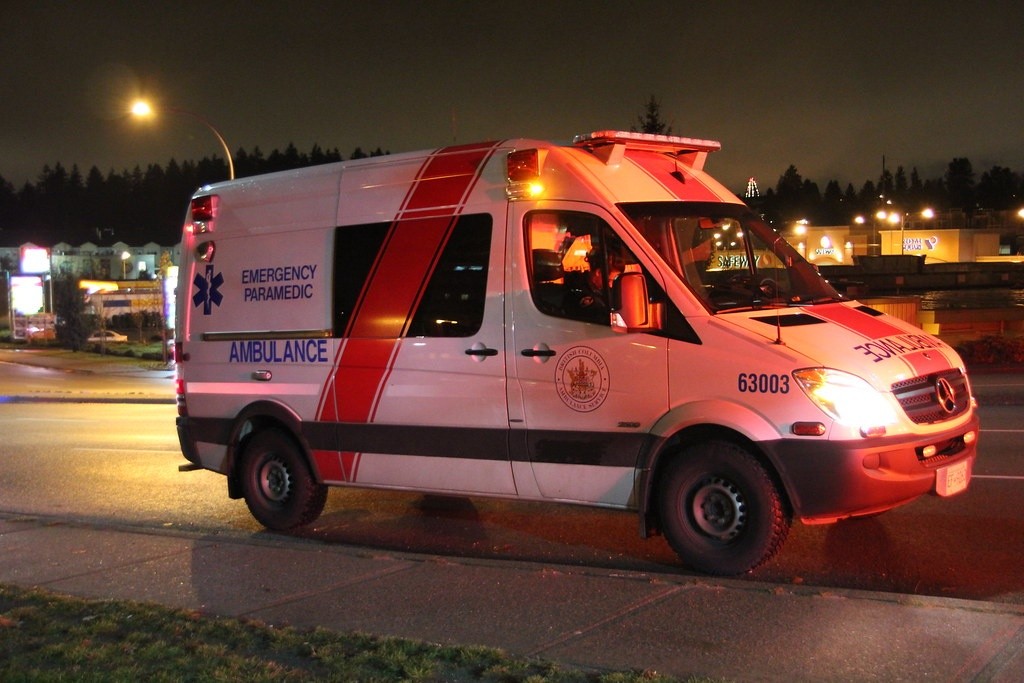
[567,245,626,325]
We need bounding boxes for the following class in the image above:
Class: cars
[86,329,128,344]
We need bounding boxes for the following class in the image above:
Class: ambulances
[171,129,979,579]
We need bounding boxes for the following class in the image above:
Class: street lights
[120,250,131,280]
[901,208,932,256]
[793,221,807,259]
[132,101,234,181]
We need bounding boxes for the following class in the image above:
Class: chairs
[532,247,583,320]
